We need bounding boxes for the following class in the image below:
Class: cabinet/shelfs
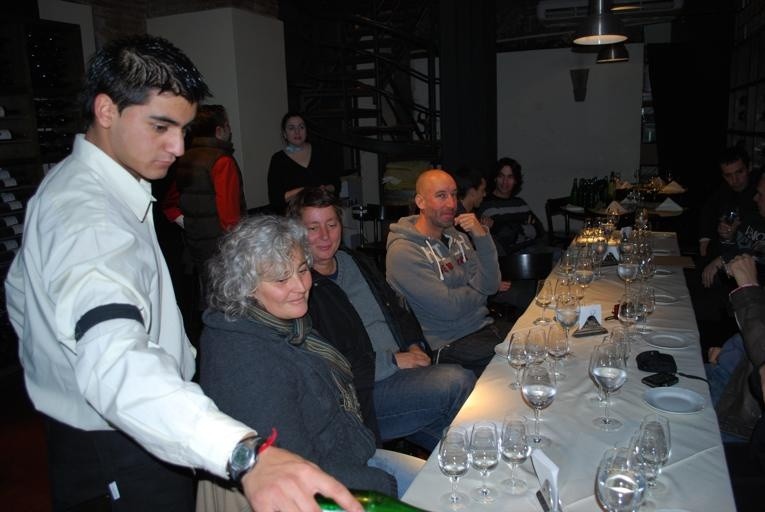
[0,0,83,421]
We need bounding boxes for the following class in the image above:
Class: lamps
[595,42,629,63]
[572,0,630,47]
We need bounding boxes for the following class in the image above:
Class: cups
[635,192,641,200]
[602,335,623,368]
[626,192,635,201]
[568,273,585,300]
[595,449,650,512]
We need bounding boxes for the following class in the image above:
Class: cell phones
[641,373,678,389]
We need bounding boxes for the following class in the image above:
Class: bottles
[314,488,431,512]
[570,172,618,208]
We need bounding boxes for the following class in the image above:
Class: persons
[451,165,543,316]
[382,169,519,374]
[703,253,765,447]
[481,158,564,262]
[697,144,765,260]
[275,187,478,457]
[267,112,343,212]
[4,36,364,511]
[183,105,248,251]
[195,216,429,497]
[692,166,765,360]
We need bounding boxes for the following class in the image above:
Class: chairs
[546,196,573,243]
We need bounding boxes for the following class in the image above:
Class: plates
[641,387,705,414]
[650,295,680,305]
[654,269,673,276]
[643,331,691,350]
[651,250,672,257]
[451,421,504,456]
[495,343,511,359]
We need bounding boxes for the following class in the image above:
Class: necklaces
[289,145,305,152]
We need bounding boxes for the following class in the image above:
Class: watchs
[229,434,271,491]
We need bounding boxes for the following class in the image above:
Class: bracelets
[720,256,727,268]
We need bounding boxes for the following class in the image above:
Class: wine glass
[553,215,656,287]
[639,286,657,334]
[437,425,470,509]
[532,280,554,326]
[637,414,670,494]
[631,292,645,338]
[592,343,627,430]
[666,168,673,184]
[553,278,571,321]
[520,353,557,449]
[617,294,638,342]
[633,160,641,182]
[610,326,632,383]
[499,415,532,496]
[720,206,742,245]
[588,345,613,405]
[546,323,570,380]
[628,430,663,512]
[524,328,548,377]
[556,293,581,353]
[508,332,526,390]
[617,254,637,297]
[468,423,501,504]
